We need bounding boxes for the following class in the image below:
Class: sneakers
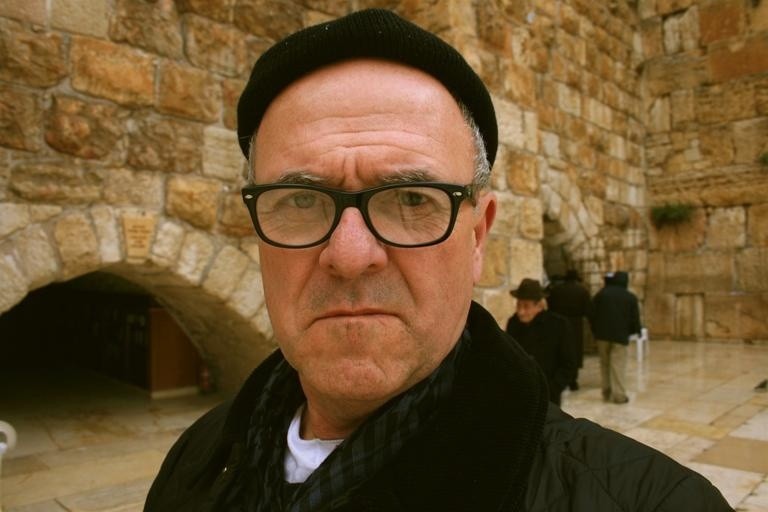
[568,382,629,404]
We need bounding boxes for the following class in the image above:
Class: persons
[506,268,642,409]
[143,8,735,512]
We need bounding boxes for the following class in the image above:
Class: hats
[510,278,550,301]
[236,8,499,172]
[559,268,629,287]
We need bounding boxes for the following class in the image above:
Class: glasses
[241,177,474,250]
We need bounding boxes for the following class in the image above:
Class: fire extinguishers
[201,368,212,392]
